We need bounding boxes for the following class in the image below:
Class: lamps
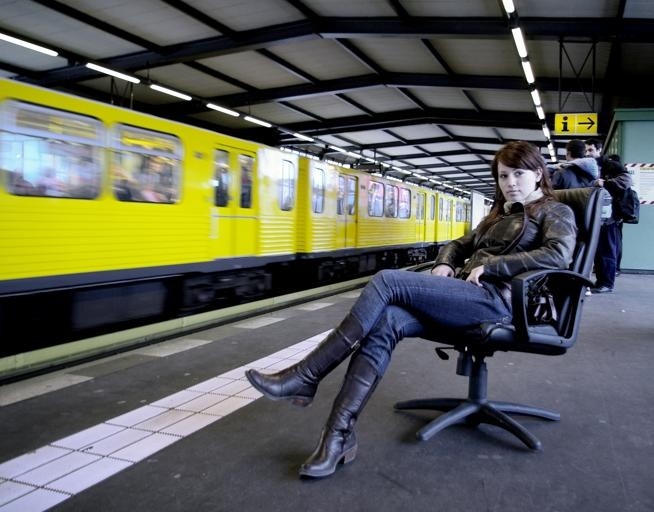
[242,115,273,129]
[279,128,471,197]
[147,83,194,103]
[2,29,58,57]
[84,62,140,85]
[205,102,241,119]
[503,0,557,164]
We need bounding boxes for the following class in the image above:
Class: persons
[583,137,632,294]
[242,140,580,482]
[552,140,599,190]
[0,104,251,209]
[592,154,625,277]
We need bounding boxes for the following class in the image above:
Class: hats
[608,155,618,161]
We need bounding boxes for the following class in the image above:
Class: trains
[0,75,495,359]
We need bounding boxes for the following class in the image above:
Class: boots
[243,309,366,406]
[295,350,381,481]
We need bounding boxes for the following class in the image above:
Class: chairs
[394,186,604,451]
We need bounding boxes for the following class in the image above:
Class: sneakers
[584,266,622,296]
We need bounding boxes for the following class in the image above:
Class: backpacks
[620,185,641,225]
[561,161,614,227]
